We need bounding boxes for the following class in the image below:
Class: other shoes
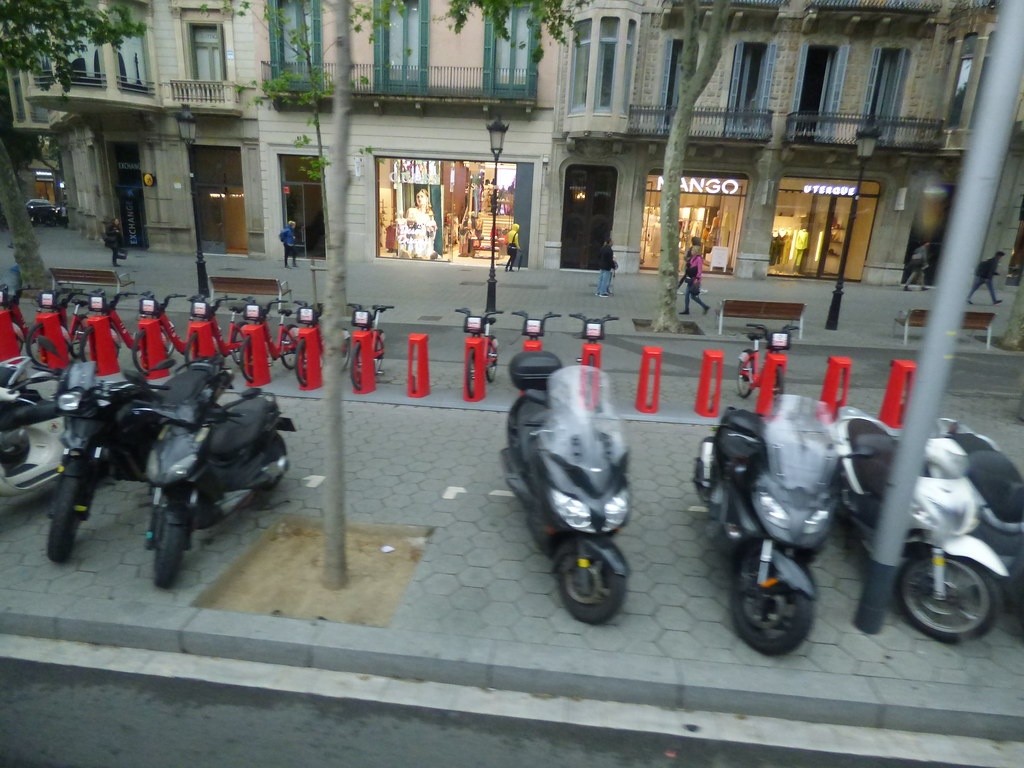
[509,269,514,272]
[965,300,973,303]
[993,299,1001,304]
[595,292,609,298]
[703,306,710,314]
[679,309,689,314]
[504,266,508,271]
[113,264,121,267]
[921,287,928,290]
[903,286,912,291]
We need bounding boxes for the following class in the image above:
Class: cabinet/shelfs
[829,227,844,260]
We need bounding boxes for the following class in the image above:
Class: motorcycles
[36,332,169,563]
[1,352,72,492]
[495,346,638,627]
[133,335,297,590]
[831,405,1022,643]
[691,389,833,657]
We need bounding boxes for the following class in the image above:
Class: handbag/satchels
[507,243,518,255]
[102,233,117,243]
[685,265,698,282]
[117,248,128,260]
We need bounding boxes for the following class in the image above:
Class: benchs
[714,298,807,340]
[208,276,292,309]
[49,267,136,295]
[892,308,997,350]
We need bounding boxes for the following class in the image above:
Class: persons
[903,240,930,291]
[105,217,123,268]
[405,189,437,260]
[967,251,1006,305]
[283,221,297,268]
[677,238,710,316]
[769,225,809,266]
[505,224,521,272]
[596,238,618,298]
[62,203,69,228]
[467,211,482,254]
[709,210,720,247]
[650,216,662,257]
[480,178,498,212]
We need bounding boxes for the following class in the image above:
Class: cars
[27,198,58,227]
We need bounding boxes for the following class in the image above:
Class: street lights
[482,113,509,314]
[824,113,885,329]
[175,104,214,297]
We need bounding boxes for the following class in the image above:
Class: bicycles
[238,295,295,384]
[736,320,804,402]
[0,284,29,350]
[33,285,238,369]
[454,303,498,396]
[571,308,617,399]
[509,310,560,387]
[291,297,326,389]
[347,300,395,391]
